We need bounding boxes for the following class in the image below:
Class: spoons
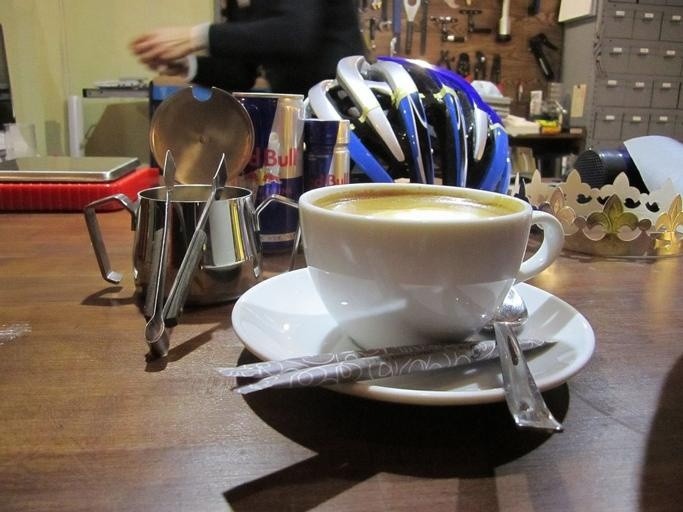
[484,286,565,434]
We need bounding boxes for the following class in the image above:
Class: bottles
[83,84,300,307]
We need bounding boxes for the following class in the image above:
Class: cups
[296,183,566,349]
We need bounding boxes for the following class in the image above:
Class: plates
[231,266,596,406]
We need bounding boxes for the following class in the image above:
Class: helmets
[304,55,511,195]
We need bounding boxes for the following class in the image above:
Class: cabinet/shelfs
[560,0,682,152]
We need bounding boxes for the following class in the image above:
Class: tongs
[145,149,229,357]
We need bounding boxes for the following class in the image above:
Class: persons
[129,0,372,102]
